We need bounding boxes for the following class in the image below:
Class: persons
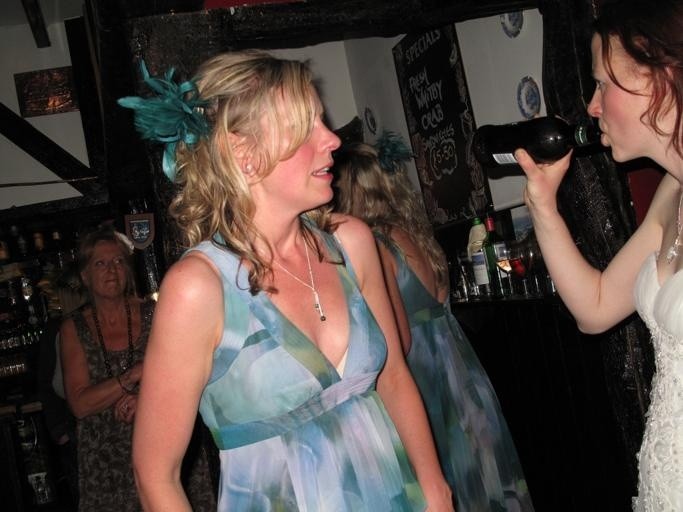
[59,231,218,511]
[514,0,683,511]
[337,140,535,510]
[117,51,458,511]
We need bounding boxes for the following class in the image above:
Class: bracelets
[116,374,130,394]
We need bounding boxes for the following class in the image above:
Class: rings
[124,403,130,409]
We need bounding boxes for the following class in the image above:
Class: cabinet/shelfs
[0,207,128,512]
[448,275,640,512]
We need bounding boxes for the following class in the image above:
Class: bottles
[466,216,515,299]
[470,115,604,179]
[0,228,68,351]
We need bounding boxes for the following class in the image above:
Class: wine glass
[490,244,547,297]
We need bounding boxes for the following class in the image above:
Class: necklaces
[92,296,135,378]
[264,237,328,322]
[666,192,683,261]
